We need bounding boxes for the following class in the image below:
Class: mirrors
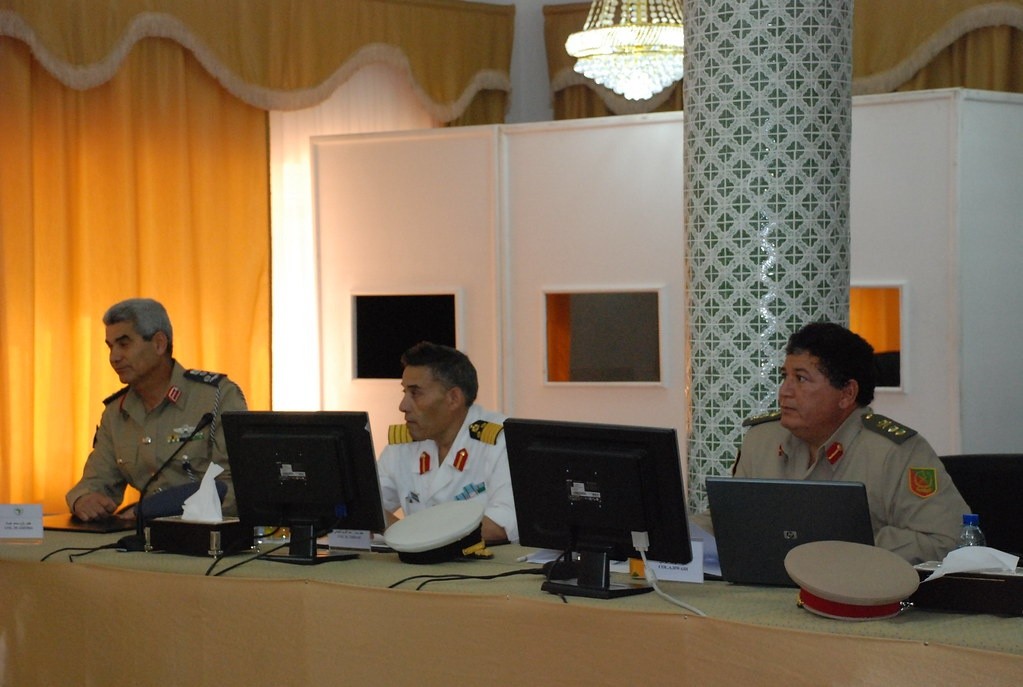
[544,288,666,385]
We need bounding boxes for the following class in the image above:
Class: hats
[384,500,495,563]
[133,479,227,529]
[785,541,922,621]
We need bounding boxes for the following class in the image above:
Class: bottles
[954,514,987,549]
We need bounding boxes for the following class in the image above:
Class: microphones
[117,412,215,552]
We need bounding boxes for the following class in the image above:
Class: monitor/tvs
[221,411,385,534]
[503,417,693,564]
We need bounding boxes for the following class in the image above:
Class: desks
[2,507,1023,687]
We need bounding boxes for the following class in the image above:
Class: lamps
[564,0,685,99]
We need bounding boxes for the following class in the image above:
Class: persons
[681,321,980,567]
[371,341,521,547]
[66,298,248,523]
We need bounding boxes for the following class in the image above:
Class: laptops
[704,478,876,588]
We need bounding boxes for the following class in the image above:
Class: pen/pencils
[517,552,535,563]
